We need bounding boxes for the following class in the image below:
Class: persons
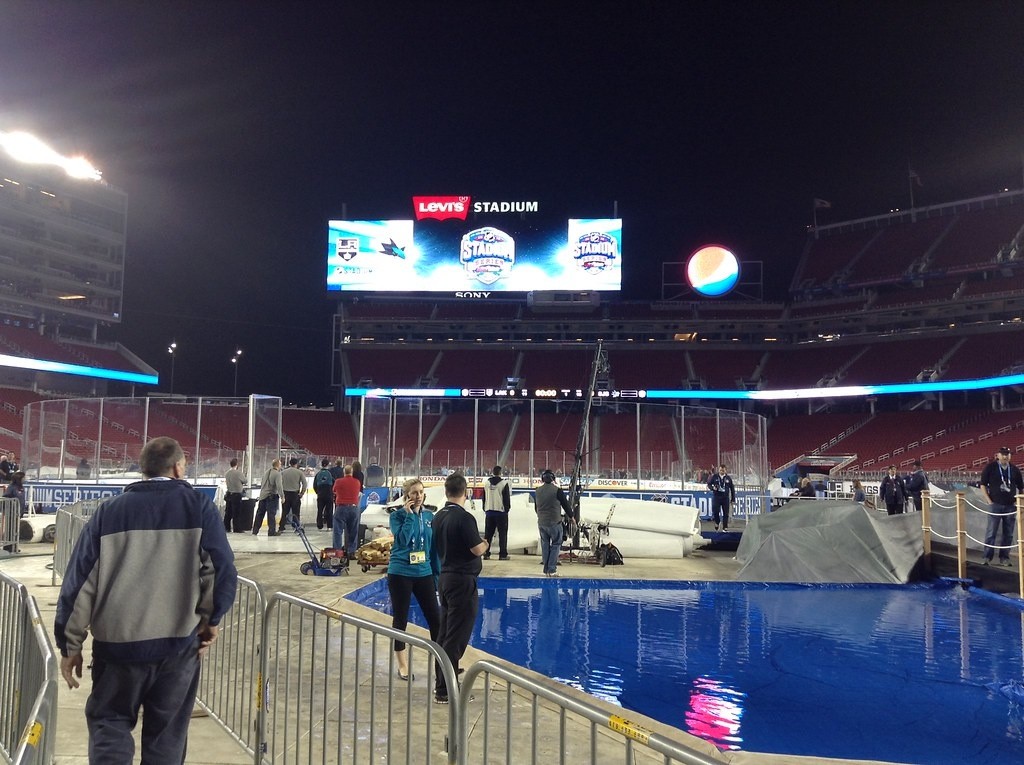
[438,465,638,479]
[279,458,307,531]
[533,470,577,578]
[790,477,828,500]
[54,436,238,765]
[852,480,866,505]
[75,459,90,480]
[388,478,464,681]
[695,464,737,532]
[313,456,386,560]
[252,458,286,535]
[980,446,1024,566]
[0,452,26,552]
[431,474,489,704]
[220,458,247,533]
[481,466,512,561]
[879,461,930,516]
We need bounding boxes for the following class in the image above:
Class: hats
[999,447,1011,456]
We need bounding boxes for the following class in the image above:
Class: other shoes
[499,556,510,560]
[1000,557,1013,566]
[722,529,729,532]
[546,572,563,576]
[434,694,475,704]
[398,668,415,681]
[714,521,721,532]
[484,556,490,560]
[980,557,991,565]
[268,532,281,536]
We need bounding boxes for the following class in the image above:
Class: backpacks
[596,542,624,565]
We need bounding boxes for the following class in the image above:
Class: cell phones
[405,495,414,511]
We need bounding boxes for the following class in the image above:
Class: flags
[908,161,924,188]
[813,197,833,208]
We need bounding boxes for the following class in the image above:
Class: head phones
[541,471,555,482]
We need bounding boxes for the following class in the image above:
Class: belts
[336,503,357,506]
